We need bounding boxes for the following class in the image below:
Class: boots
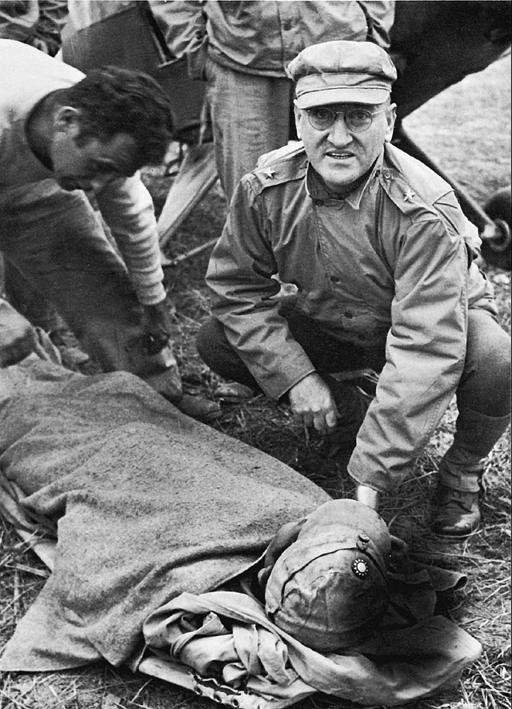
[434,404,512,542]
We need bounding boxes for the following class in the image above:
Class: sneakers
[166,391,220,422]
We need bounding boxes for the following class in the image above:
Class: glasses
[301,105,392,132]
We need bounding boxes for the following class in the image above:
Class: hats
[265,498,393,653]
[287,40,399,110]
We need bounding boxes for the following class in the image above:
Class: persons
[251,497,390,655]
[2,38,224,423]
[195,39,512,541]
[149,1,398,298]
